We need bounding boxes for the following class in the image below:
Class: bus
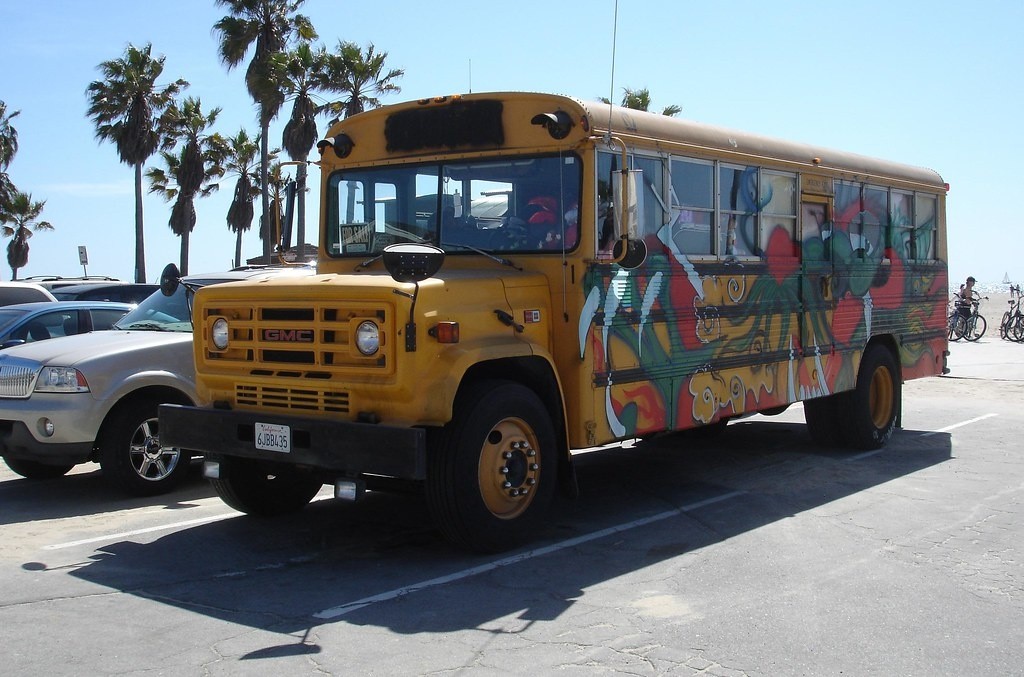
[159,93,951,560]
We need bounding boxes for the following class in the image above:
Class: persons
[1009,284,1020,298]
[948,276,978,341]
[518,166,613,250]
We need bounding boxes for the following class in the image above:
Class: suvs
[0,263,269,498]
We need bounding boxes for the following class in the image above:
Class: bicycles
[998,288,1024,343]
[946,290,990,343]
